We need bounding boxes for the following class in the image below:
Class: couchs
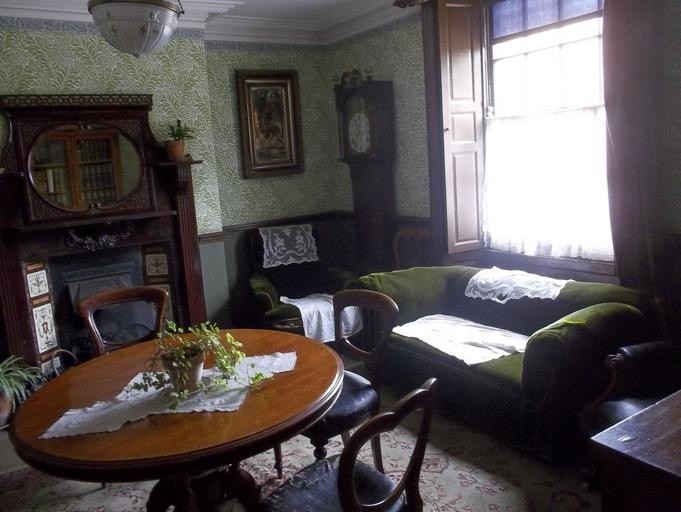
[344,264,647,459]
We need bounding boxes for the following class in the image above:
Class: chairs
[76,285,169,356]
[245,222,365,351]
[260,289,438,511]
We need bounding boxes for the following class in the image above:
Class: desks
[590,385,680,511]
[8,328,345,511]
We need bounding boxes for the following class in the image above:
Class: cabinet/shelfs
[0,94,209,374]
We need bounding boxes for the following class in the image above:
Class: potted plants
[163,118,195,162]
[0,356,48,432]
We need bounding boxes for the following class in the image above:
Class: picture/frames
[234,68,306,180]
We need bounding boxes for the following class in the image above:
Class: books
[33,138,117,211]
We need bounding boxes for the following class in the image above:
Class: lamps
[86,0,185,59]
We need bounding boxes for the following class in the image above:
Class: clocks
[348,114,372,153]
